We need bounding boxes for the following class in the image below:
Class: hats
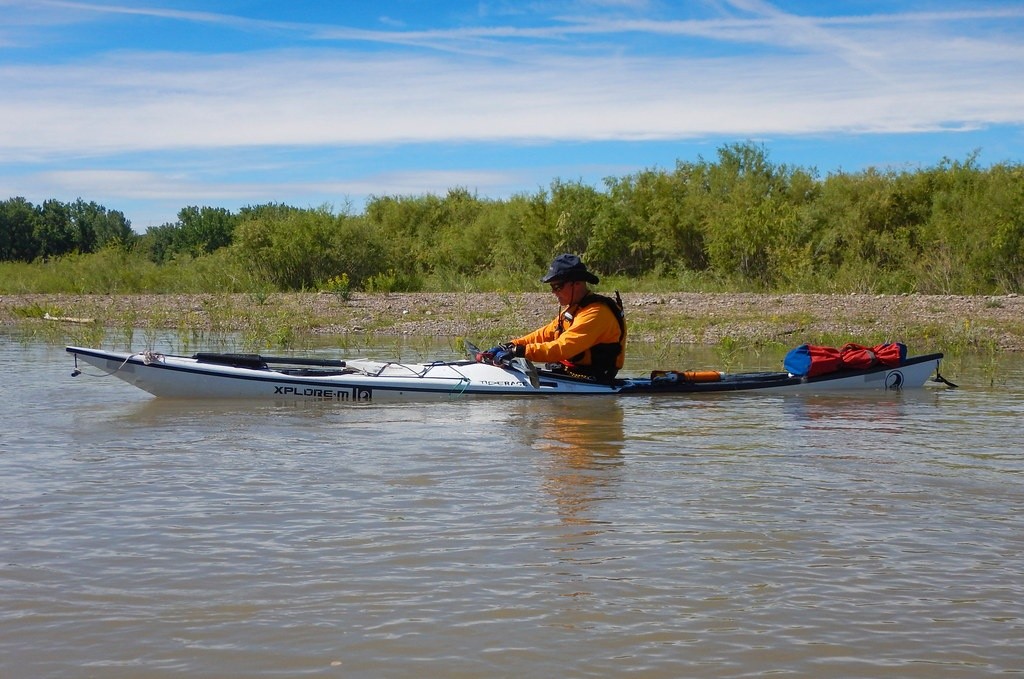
[542,255,600,285]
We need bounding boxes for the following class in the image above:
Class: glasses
[550,281,569,291]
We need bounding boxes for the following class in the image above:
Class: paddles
[454,337,540,390]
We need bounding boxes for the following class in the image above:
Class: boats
[65,342,944,401]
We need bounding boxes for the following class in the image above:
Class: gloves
[476,346,505,362]
[493,350,514,365]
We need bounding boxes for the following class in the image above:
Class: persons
[475,254,628,380]
